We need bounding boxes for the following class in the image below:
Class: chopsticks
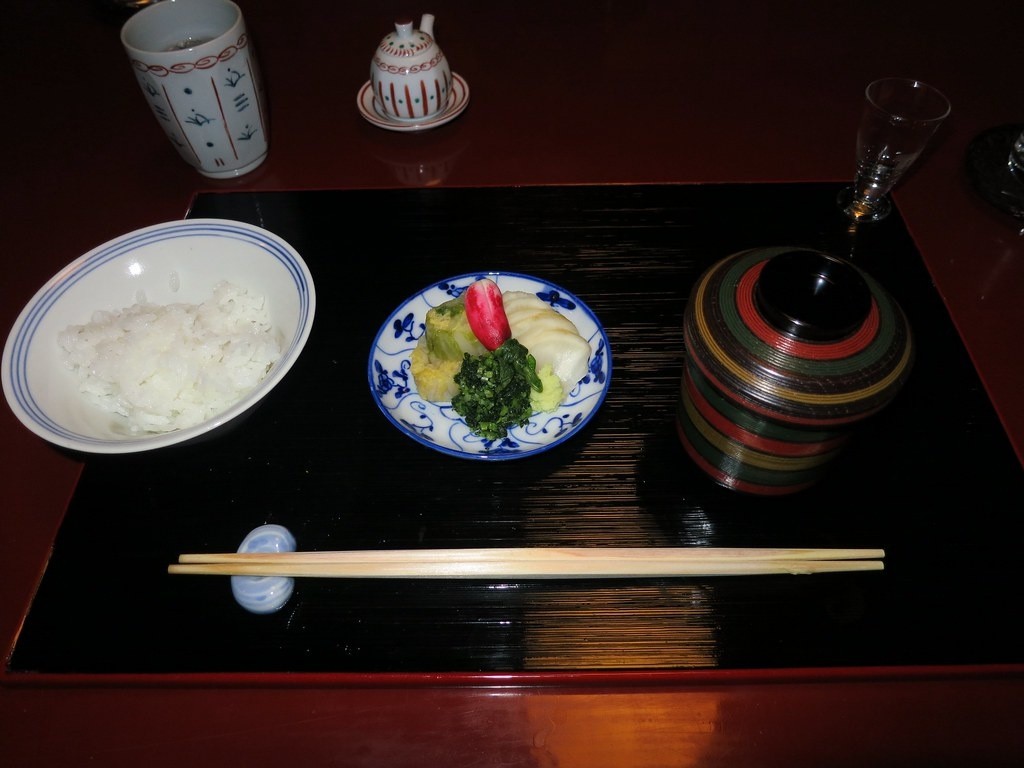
[167,547,888,578]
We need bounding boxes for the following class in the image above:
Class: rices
[57,281,280,434]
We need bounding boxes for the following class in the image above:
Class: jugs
[368,14,453,122]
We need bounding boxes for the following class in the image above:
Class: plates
[367,273,612,462]
[357,72,470,131]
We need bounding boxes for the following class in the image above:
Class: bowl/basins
[1,219,318,455]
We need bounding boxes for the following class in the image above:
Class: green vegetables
[451,341,543,440]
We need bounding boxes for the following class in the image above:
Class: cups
[676,246,915,497]
[119,0,268,179]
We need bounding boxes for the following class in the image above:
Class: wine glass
[837,78,952,221]
[1008,132,1024,239]
